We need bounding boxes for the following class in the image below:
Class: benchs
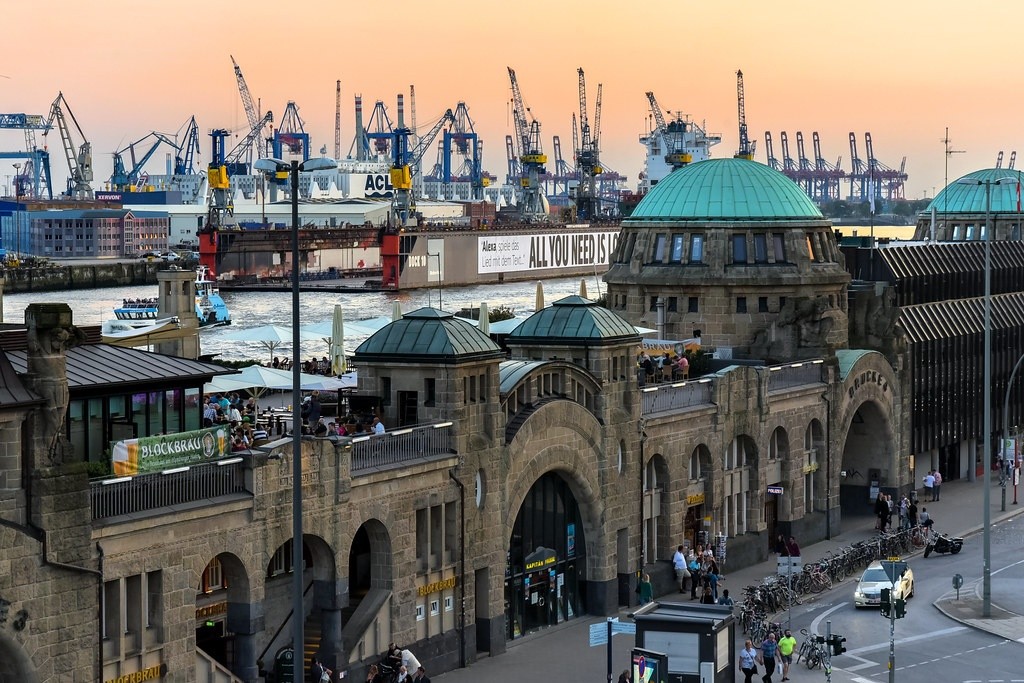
[257,407,293,433]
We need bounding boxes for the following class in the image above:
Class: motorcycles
[923,529,964,558]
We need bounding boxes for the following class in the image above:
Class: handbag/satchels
[921,476,929,487]
[319,666,330,683]
[777,662,783,675]
[752,663,759,675]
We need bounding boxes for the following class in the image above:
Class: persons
[618,670,632,683]
[739,640,763,683]
[673,543,734,606]
[778,628,797,682]
[637,351,688,387]
[311,644,431,683]
[759,632,781,683]
[202,390,385,451]
[874,468,942,537]
[634,573,653,606]
[997,453,1022,487]
[788,536,800,567]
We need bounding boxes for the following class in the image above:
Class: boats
[111,268,232,326]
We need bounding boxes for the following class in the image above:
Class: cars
[854,559,915,611]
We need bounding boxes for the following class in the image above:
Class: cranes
[0,54,1016,221]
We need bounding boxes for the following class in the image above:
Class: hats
[392,649,401,658]
[641,573,650,582]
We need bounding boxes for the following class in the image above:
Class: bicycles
[735,523,928,671]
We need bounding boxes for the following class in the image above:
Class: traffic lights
[833,635,846,656]
[896,599,907,619]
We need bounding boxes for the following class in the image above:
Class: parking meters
[952,574,963,601]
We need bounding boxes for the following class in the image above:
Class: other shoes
[691,595,699,600]
[785,678,790,680]
[781,678,785,682]
[680,590,686,594]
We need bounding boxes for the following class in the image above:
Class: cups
[114,439,138,476]
[217,431,225,456]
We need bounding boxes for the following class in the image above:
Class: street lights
[429,251,441,311]
[252,158,305,683]
[956,175,1019,616]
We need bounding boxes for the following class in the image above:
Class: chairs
[647,365,689,383]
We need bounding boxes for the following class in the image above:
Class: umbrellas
[167,313,529,427]
[392,300,402,323]
[536,279,545,313]
[633,325,659,335]
[479,301,490,338]
[331,304,347,377]
[581,278,587,300]
